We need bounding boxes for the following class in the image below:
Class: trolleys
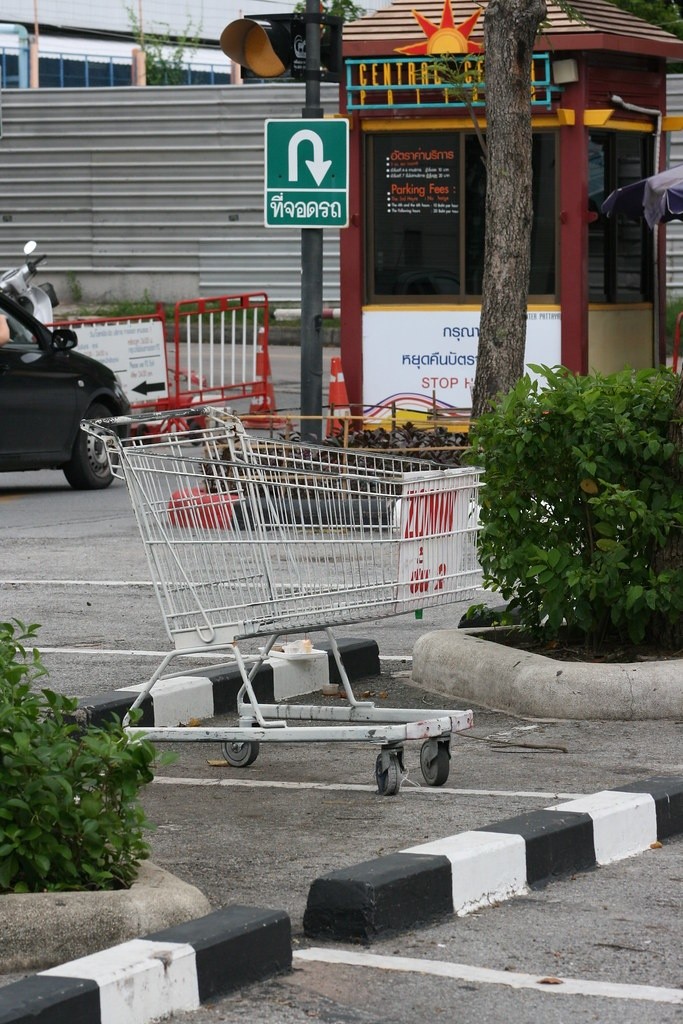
[78,402,492,795]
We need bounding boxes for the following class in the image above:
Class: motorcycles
[1,240,59,343]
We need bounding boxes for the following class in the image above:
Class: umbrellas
[601,162,683,229]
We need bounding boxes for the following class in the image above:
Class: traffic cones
[243,328,287,426]
[325,357,355,437]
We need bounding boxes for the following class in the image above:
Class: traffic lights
[219,11,341,85]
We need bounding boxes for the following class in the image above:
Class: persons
[0,314,10,346]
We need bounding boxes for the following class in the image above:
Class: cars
[1,290,131,492]
